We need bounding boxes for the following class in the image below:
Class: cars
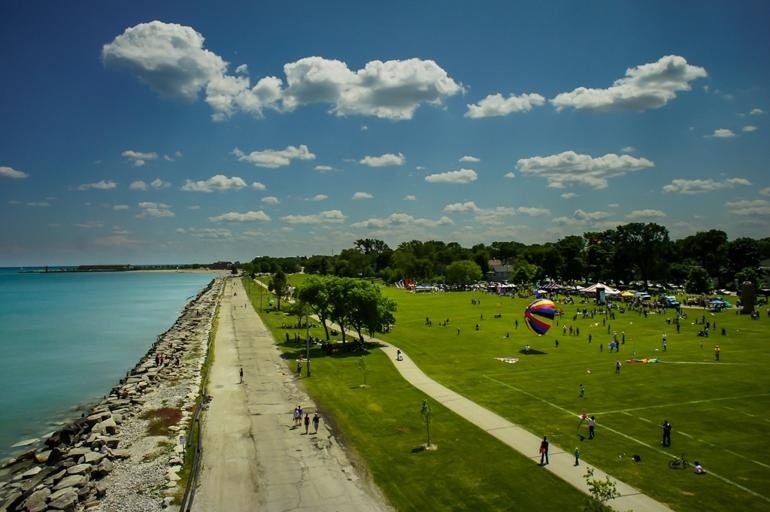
[716,289,731,295]
[588,277,684,290]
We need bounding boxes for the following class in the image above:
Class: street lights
[304,302,312,376]
[260,277,265,314]
[244,271,251,298]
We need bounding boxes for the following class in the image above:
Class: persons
[695,461,706,474]
[397,350,403,361]
[662,421,671,447]
[575,447,580,465]
[304,413,310,433]
[293,408,299,425]
[577,413,588,431]
[312,413,321,433]
[297,406,302,425]
[580,384,584,396]
[240,368,243,383]
[539,436,549,464]
[286,333,364,360]
[585,416,596,439]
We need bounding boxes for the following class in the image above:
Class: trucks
[634,292,652,304]
[659,295,679,308]
